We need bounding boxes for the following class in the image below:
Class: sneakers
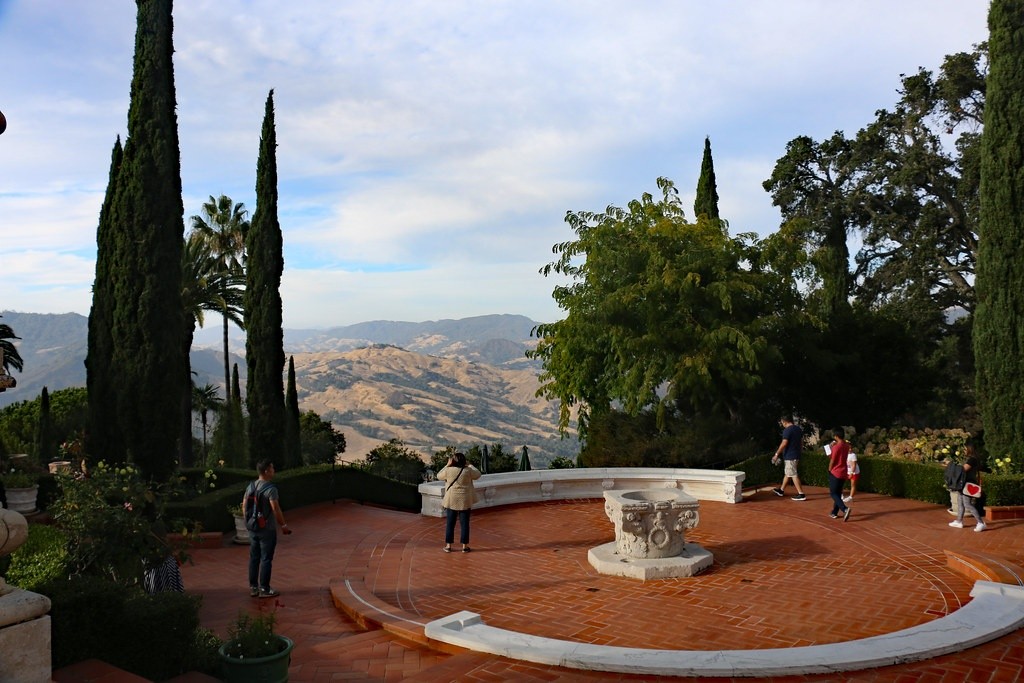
[773,487,784,496]
[259,587,279,597]
[828,510,837,519]
[841,494,843,499]
[791,494,806,500]
[974,521,989,532]
[949,520,963,528]
[462,547,471,553]
[443,546,451,552]
[843,508,851,522]
[843,497,853,503]
[249,587,258,596]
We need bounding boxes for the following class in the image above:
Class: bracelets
[775,453,779,457]
[281,523,287,527]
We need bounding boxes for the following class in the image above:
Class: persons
[828,426,860,523]
[243,459,289,598]
[772,415,806,500]
[948,441,987,532]
[437,453,481,553]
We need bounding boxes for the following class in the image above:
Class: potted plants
[226,503,250,540]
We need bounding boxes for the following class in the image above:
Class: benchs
[424,580,1024,679]
[418,468,745,518]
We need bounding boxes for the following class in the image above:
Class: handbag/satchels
[962,482,982,498]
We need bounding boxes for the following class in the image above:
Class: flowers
[224,598,286,659]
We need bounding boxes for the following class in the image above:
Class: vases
[218,635,293,683]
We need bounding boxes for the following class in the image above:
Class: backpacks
[244,481,275,532]
